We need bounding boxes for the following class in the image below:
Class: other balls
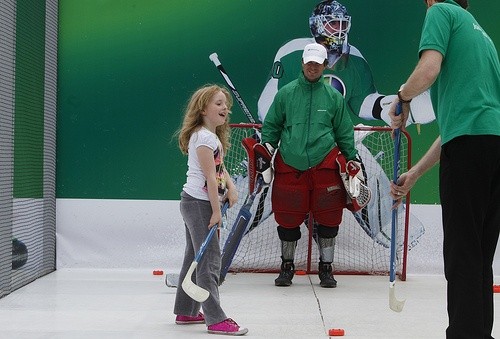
[152,270,164,276]
[493,285,500,292]
[296,270,306,275]
[329,328,344,337]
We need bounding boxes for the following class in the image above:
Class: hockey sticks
[389,103,406,313]
[209,52,262,140]
[166,176,264,288]
[181,200,230,303]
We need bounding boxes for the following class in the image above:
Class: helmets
[309,1,351,50]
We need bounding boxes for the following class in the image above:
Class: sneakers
[208,318,248,335]
[175,312,206,324]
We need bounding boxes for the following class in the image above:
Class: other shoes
[274,256,295,286]
[319,256,337,287]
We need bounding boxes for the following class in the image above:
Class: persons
[215,0,424,254]
[258,43,360,289]
[389,0,500,339]
[174,84,248,336]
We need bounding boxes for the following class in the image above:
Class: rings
[397,192,403,196]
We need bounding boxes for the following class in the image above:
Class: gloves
[252,142,275,194]
[345,160,365,198]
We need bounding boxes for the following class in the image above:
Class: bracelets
[397,88,412,103]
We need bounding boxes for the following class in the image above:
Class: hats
[302,42,327,65]
